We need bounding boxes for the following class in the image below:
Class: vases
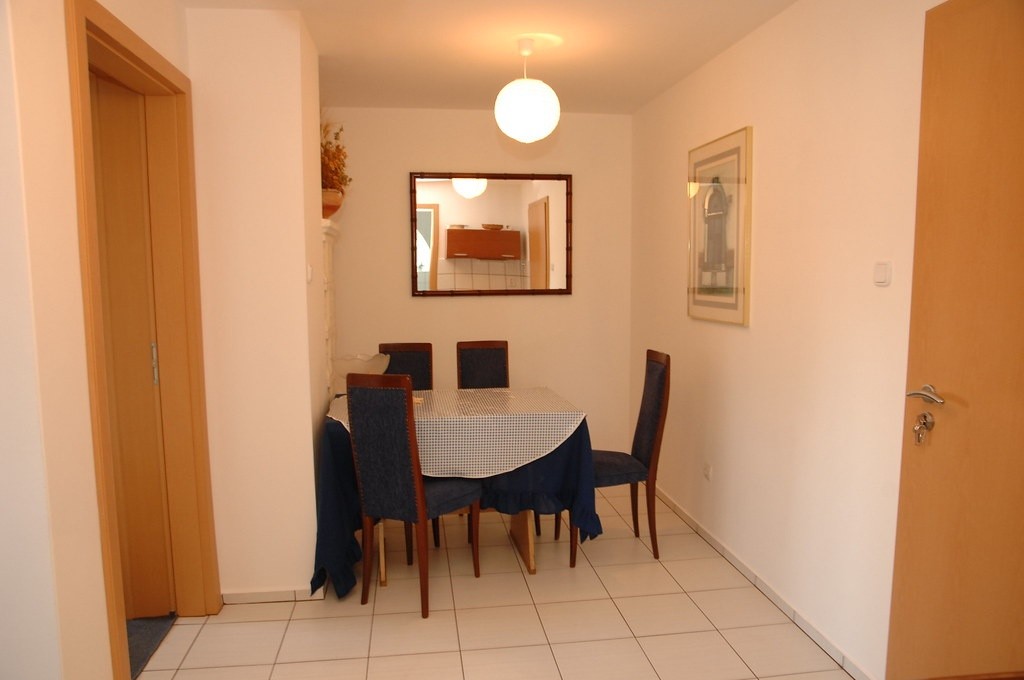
[322,189,344,219]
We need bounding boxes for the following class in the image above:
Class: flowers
[320,105,353,196]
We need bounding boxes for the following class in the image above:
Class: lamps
[495,32,563,143]
[452,178,488,198]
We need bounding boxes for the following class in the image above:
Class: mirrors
[410,172,574,296]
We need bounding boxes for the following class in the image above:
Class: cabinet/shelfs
[444,229,521,260]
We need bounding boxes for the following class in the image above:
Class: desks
[310,386,603,601]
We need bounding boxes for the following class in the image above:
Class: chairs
[554,349,671,568]
[346,340,541,619]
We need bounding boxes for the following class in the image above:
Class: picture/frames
[686,126,753,328]
